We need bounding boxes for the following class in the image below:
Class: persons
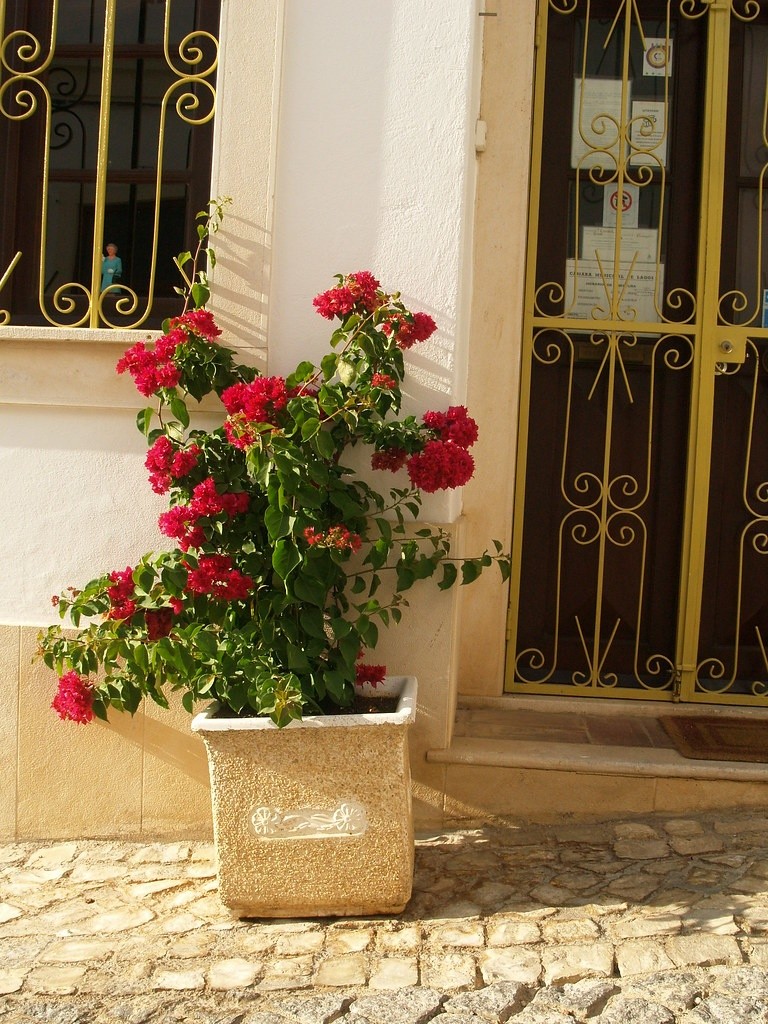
[102,244,122,294]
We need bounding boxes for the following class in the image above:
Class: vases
[192,675,418,918]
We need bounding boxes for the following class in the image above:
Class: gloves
[101,254,105,262]
[108,269,114,274]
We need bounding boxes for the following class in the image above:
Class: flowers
[36,273,511,735]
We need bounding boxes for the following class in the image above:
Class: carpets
[658,715,768,762]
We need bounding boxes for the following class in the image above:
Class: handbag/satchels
[112,269,121,284]
